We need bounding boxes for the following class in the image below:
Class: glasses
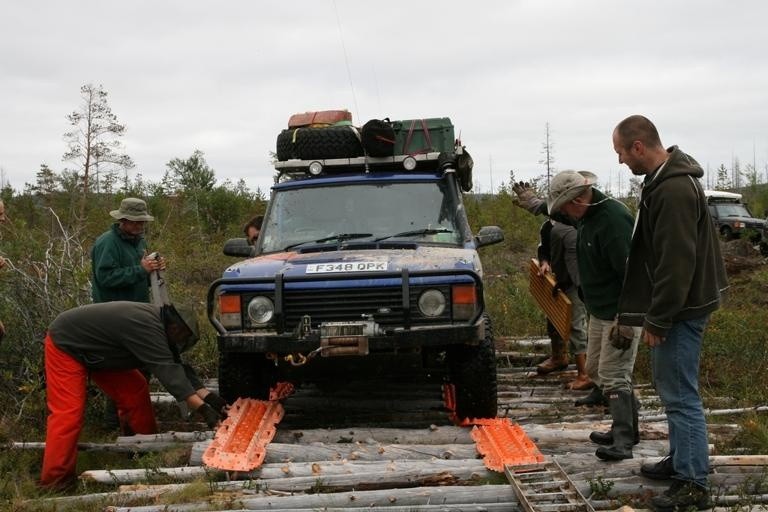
[247,233,258,246]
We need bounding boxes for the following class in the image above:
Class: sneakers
[649,480,712,512]
[640,451,679,480]
[575,385,608,407]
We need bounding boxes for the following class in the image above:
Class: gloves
[551,280,572,302]
[577,284,585,305]
[511,180,544,216]
[203,392,228,413]
[608,313,635,351]
[195,403,224,430]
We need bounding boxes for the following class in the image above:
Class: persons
[510,170,638,462]
[613,116,731,510]
[243,216,267,249]
[40,303,227,490]
[535,220,590,389]
[90,197,165,301]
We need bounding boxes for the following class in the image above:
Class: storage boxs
[391,117,455,158]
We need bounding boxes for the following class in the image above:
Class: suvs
[206,146,505,420]
[706,195,767,243]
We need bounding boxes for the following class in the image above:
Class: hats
[109,197,155,221]
[546,170,598,217]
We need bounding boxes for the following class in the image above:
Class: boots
[590,391,640,445]
[535,342,569,374]
[595,389,635,462]
[564,353,594,390]
